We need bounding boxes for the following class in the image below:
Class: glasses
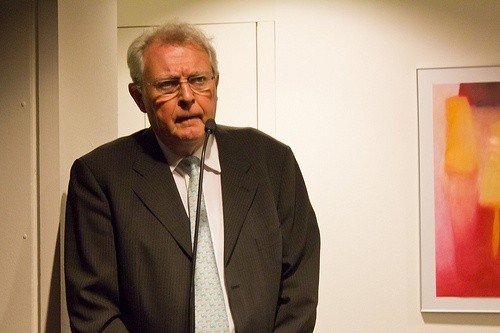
[145,73,216,93]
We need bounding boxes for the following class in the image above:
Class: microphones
[188,117,217,333]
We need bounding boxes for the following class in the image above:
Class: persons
[64,22,321,333]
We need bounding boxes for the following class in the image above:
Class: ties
[184,155,230,332]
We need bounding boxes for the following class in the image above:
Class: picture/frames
[415,61,500,314]
